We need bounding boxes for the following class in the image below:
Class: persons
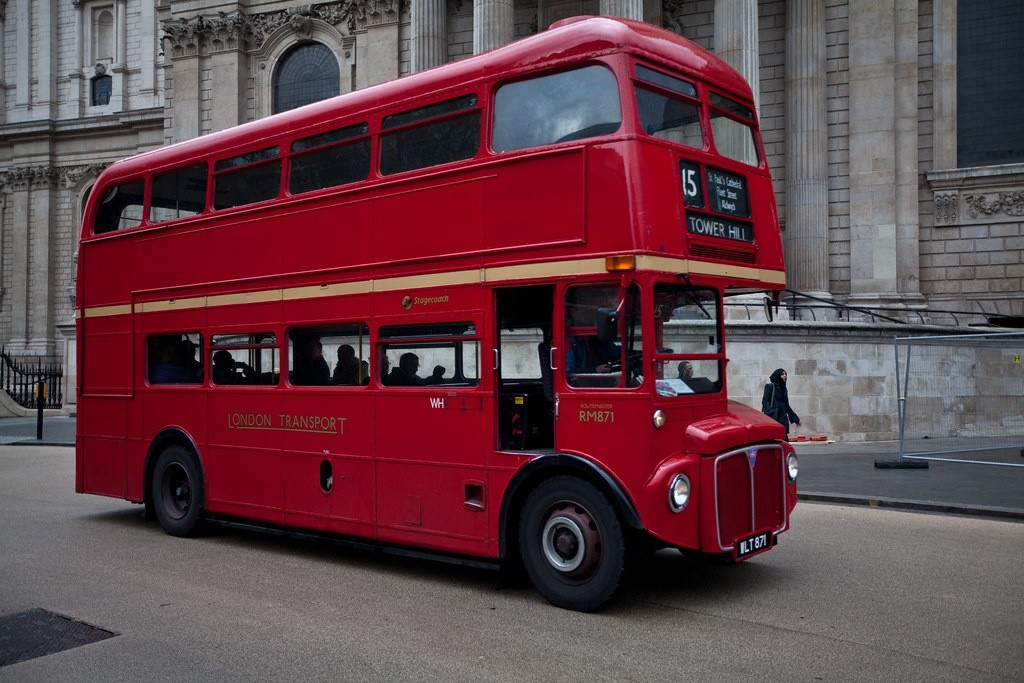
[176,339,203,383]
[332,345,365,386]
[212,350,259,385]
[389,352,445,386]
[290,340,330,386]
[362,356,395,386]
[566,309,642,374]
[762,369,802,443]
[678,362,694,379]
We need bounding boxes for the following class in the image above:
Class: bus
[73,12,801,614]
